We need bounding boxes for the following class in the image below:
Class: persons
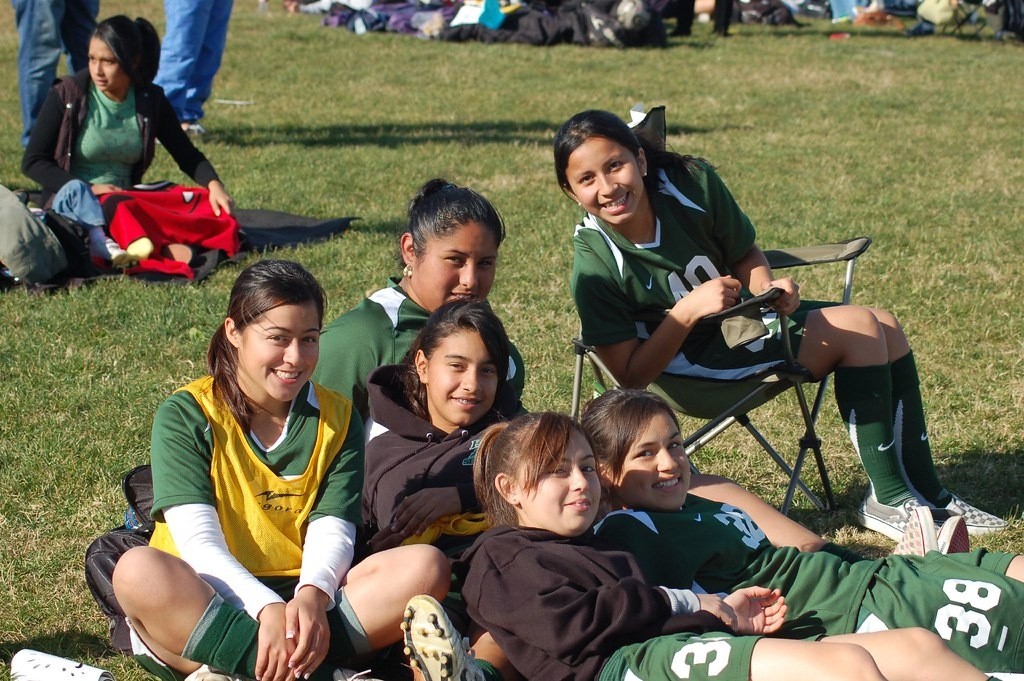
[11,0,235,148]
[667,0,734,37]
[111,180,1024,681]
[0,14,235,288]
[554,109,1010,548]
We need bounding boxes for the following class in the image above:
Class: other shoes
[161,243,193,264]
[184,663,234,681]
[930,491,1010,536]
[666,27,692,38]
[935,515,970,556]
[184,123,205,138]
[893,506,938,559]
[333,667,383,681]
[857,487,941,542]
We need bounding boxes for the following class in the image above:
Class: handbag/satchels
[84,465,156,652]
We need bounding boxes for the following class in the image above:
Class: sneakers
[399,595,486,681]
[89,236,128,263]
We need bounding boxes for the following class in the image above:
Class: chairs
[571,103,873,518]
